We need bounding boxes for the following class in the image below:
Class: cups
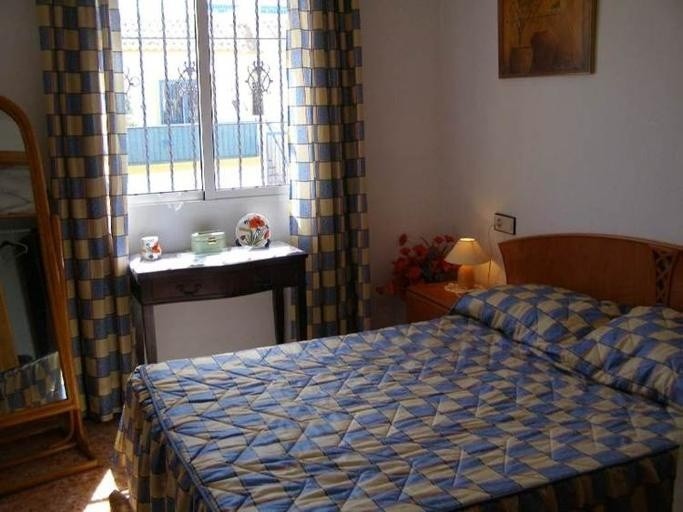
[140,236,162,262]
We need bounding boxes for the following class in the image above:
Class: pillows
[448,283,621,360]
[555,306,683,411]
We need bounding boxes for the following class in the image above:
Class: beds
[113,234,682,512]
[0,352,68,413]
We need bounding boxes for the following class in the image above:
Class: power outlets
[494,213,515,235]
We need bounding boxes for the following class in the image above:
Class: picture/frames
[498,0,597,78]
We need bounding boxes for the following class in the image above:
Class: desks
[130,240,309,364]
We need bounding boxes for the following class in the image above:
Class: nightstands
[406,282,458,322]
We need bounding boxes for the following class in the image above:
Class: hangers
[0,192,32,211]
[0,240,28,258]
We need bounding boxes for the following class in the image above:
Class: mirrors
[0,96,104,496]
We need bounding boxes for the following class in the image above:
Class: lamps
[444,238,490,294]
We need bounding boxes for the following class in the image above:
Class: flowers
[376,233,460,300]
